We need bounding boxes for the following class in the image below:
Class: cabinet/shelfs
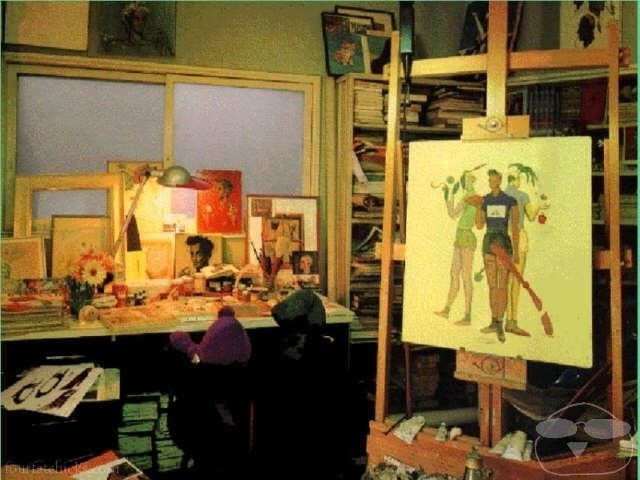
[328,65,638,411]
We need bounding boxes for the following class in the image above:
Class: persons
[99,2,175,58]
[296,252,317,276]
[176,236,215,279]
[201,175,241,233]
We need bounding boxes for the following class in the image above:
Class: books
[591,142,637,220]
[352,79,427,344]
[506,83,638,130]
[0,293,182,336]
[421,84,486,128]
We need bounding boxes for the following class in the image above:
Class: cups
[112,284,128,307]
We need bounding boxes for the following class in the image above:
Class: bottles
[462,454,483,480]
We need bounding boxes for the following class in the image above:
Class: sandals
[479,325,530,343]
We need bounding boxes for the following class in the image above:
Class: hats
[271,288,328,334]
[169,306,251,364]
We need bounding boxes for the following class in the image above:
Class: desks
[1,296,353,480]
